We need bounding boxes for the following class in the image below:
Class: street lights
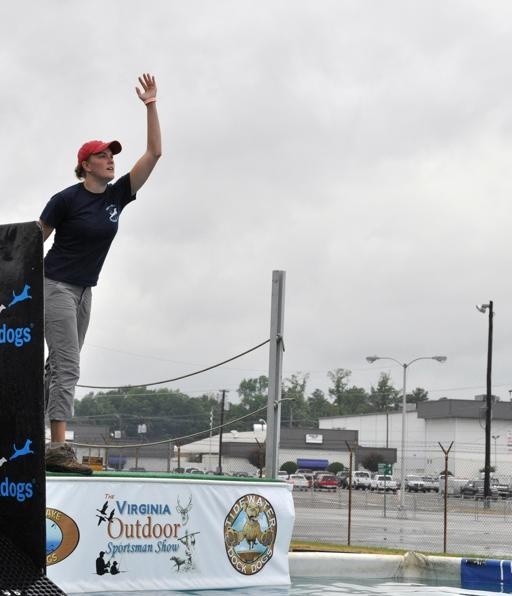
[365,355,447,521]
[476,299,494,509]
[216,388,229,476]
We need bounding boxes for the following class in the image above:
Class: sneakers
[45,443,92,475]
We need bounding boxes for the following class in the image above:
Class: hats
[77,140,122,165]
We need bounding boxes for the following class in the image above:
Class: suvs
[346,471,371,490]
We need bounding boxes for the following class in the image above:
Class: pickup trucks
[371,475,397,492]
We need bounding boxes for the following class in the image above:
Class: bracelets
[143,97,157,105]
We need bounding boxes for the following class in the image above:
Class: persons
[96,551,110,575]
[111,561,120,575]
[39,73,161,474]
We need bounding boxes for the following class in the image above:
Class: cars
[404,474,512,501]
[289,475,309,488]
[277,471,289,480]
[314,474,338,489]
[336,471,350,487]
[173,466,267,479]
[294,469,333,487]
